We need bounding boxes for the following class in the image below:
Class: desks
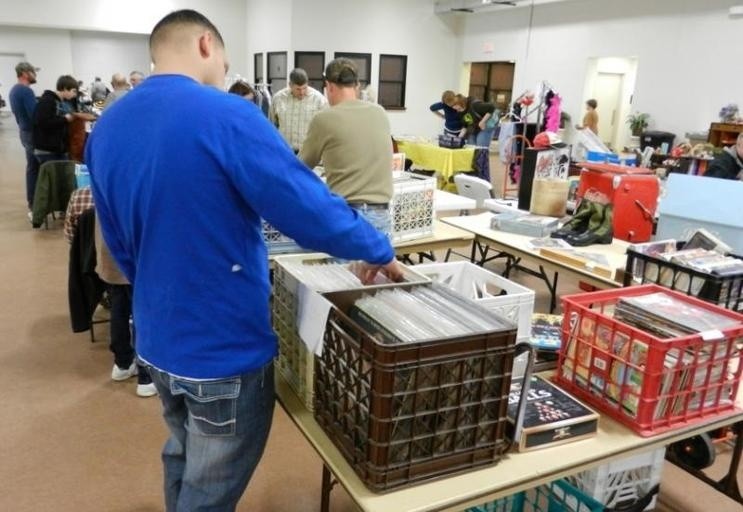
[697,121,743,176]
[399,139,485,180]
[275,369,743,512]
[441,212,629,313]
[270,219,471,297]
[433,190,477,213]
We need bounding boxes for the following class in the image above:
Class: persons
[78,81,90,101]
[449,94,499,181]
[705,132,743,180]
[129,71,145,87]
[228,80,255,102]
[95,210,159,397]
[104,73,129,103]
[583,99,598,133]
[82,10,405,511]
[34,75,78,162]
[299,57,394,264]
[430,90,468,183]
[268,68,331,155]
[9,62,42,220]
[93,77,106,100]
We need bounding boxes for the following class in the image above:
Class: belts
[348,203,388,209]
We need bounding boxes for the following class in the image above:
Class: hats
[325,58,359,84]
[534,131,567,149]
[16,63,40,73]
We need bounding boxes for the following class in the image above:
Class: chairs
[391,134,437,177]
[32,160,82,229]
[450,148,490,183]
[452,173,519,214]
[67,210,110,343]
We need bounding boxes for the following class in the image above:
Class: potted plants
[718,104,739,124]
[623,111,650,136]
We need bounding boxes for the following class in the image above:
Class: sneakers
[111,364,140,381]
[136,382,156,397]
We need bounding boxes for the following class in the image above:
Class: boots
[566,201,615,246]
[550,198,594,238]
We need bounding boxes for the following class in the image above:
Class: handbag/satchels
[486,110,500,129]
[517,143,571,213]
[528,178,570,217]
